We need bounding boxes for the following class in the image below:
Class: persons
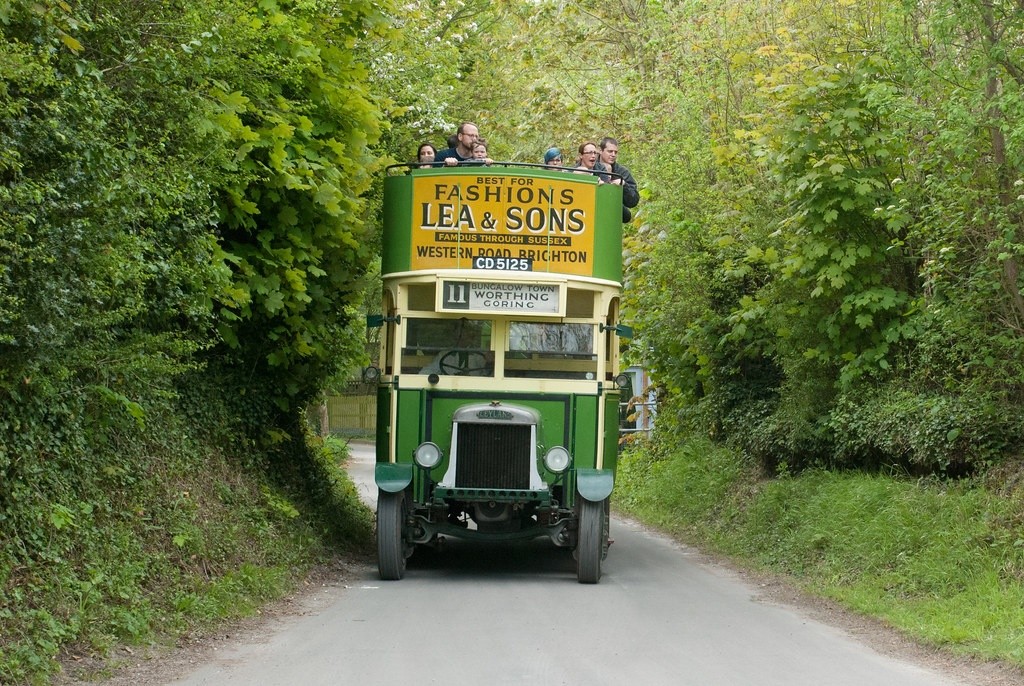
[407,122,497,168]
[540,136,639,222]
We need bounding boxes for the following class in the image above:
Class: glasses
[462,133,479,139]
[583,150,598,155]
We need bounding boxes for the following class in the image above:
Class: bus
[375,160,632,583]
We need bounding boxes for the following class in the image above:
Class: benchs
[387,356,612,381]
